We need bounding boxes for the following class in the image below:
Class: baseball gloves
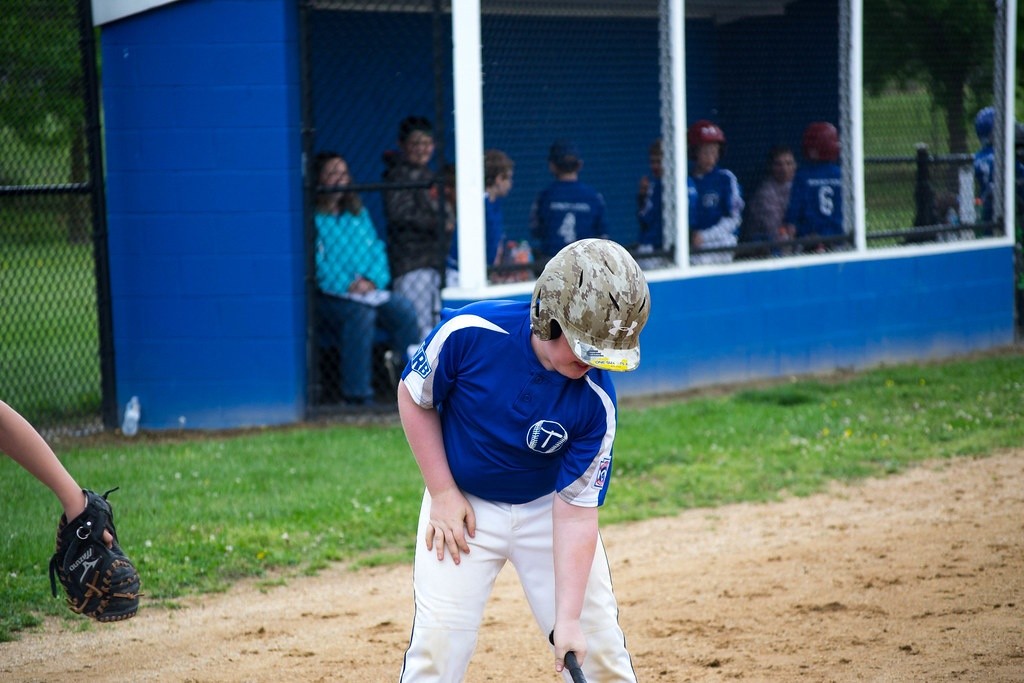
[53,488,141,624]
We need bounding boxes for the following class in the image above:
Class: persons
[381,116,456,355]
[308,151,417,414]
[902,105,1024,328]
[771,121,847,252]
[444,149,516,298]
[750,146,797,257]
[637,118,746,270]
[530,134,610,277]
[0,399,145,623]
[397,239,651,683]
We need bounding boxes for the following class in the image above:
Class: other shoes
[342,397,389,414]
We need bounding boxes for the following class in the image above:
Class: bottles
[122,396,141,436]
[506,239,531,268]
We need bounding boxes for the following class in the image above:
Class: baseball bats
[549,629,587,683]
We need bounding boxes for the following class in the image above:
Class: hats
[549,138,580,164]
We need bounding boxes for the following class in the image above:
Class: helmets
[529,238,650,372]
[803,122,840,161]
[975,106,997,146]
[688,120,724,145]
